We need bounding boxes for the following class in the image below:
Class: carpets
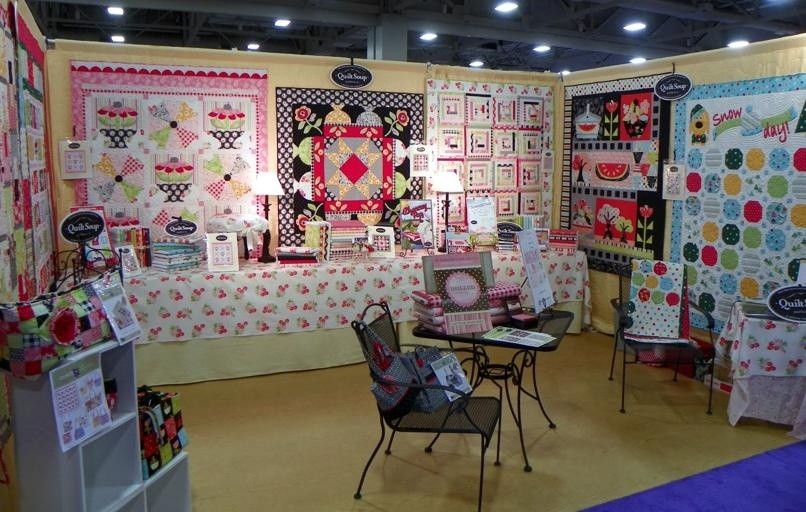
[576,440,805,512]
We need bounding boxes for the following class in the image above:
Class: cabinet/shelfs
[11,338,192,511]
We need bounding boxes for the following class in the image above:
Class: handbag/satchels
[354,320,420,421]
[136,385,190,480]
[0,268,122,383]
[104,378,118,412]
[399,346,448,413]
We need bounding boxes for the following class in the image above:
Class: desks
[413,306,575,472]
[87,244,594,391]
[734,301,805,425]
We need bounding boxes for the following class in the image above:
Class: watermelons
[596,163,630,181]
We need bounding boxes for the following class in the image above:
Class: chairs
[350,301,500,512]
[608,257,716,415]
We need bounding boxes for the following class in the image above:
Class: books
[549,228,578,256]
[152,234,202,272]
[498,229,517,251]
[111,226,155,273]
[275,220,372,265]
[408,251,522,336]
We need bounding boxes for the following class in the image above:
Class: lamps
[252,172,286,263]
[430,171,463,253]
